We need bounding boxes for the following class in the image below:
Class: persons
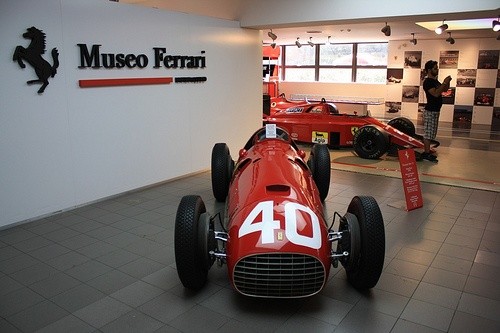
[423,60,452,140]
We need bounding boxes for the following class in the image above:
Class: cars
[174,124,385,299]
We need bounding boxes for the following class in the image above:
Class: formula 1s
[264,93,440,163]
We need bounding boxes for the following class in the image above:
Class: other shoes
[422,153,439,164]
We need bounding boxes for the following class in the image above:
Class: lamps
[446,32,455,45]
[268,28,277,40]
[492,15,500,32]
[307,37,314,48]
[271,43,277,48]
[381,22,391,36]
[410,33,417,46]
[295,37,302,48]
[435,19,448,34]
